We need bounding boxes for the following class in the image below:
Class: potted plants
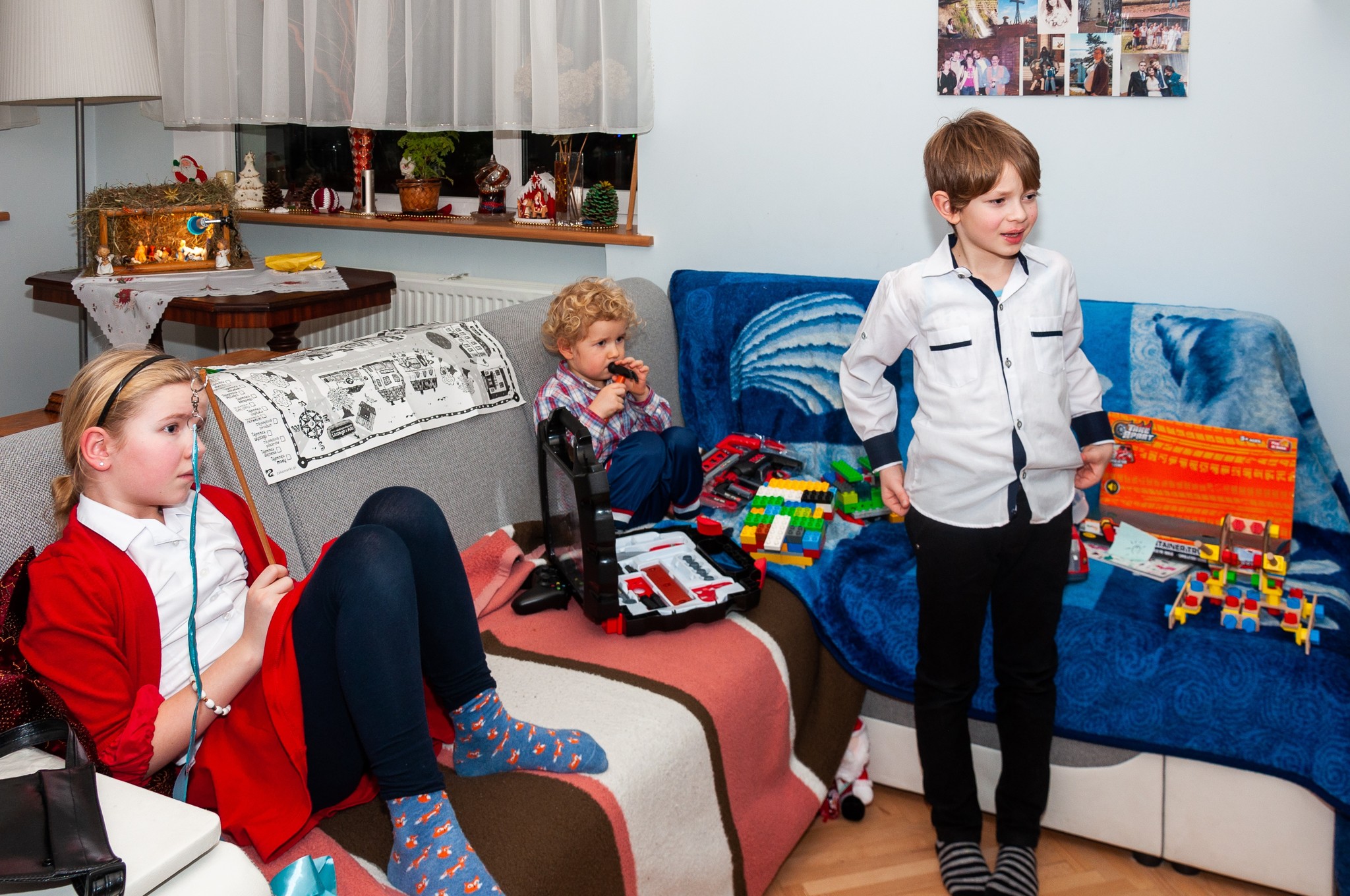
[393,129,462,215]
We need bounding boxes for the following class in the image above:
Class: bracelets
[187,674,233,716]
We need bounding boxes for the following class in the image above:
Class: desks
[27,260,396,356]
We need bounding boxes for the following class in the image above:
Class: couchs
[0,271,871,895]
[667,267,1350,896]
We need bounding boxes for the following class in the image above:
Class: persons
[937,0,1190,98]
[532,275,708,539]
[837,111,1116,890]
[93,244,115,277]
[212,238,233,271]
[16,345,609,896]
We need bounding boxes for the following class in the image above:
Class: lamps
[2,0,171,415]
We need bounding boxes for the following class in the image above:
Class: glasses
[991,59,999,61]
[1094,52,1101,55]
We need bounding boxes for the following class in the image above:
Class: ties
[1158,70,1164,88]
[1141,72,1143,80]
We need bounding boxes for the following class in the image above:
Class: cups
[555,152,584,225]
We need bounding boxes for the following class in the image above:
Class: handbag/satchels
[1,758,116,894]
[957,77,963,89]
